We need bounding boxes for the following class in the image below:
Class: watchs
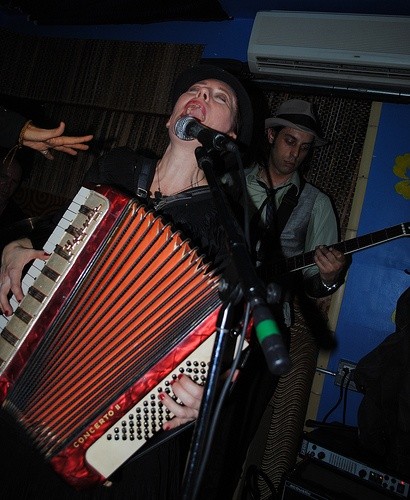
[320,279,342,292]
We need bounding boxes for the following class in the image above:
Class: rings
[41,150,48,155]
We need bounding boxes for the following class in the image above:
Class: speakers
[280,457,400,500]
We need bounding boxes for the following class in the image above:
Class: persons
[1,95,93,161]
[224,95,349,500]
[0,53,305,500]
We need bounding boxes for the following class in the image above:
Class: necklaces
[154,167,207,200]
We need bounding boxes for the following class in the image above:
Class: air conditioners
[246,9,410,99]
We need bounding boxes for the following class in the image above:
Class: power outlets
[333,358,358,393]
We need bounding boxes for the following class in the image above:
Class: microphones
[174,116,237,151]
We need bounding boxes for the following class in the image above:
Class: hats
[171,64,254,151]
[264,97,329,147]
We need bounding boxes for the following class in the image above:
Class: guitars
[287,221,410,273]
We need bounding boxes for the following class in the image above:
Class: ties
[256,165,293,230]
